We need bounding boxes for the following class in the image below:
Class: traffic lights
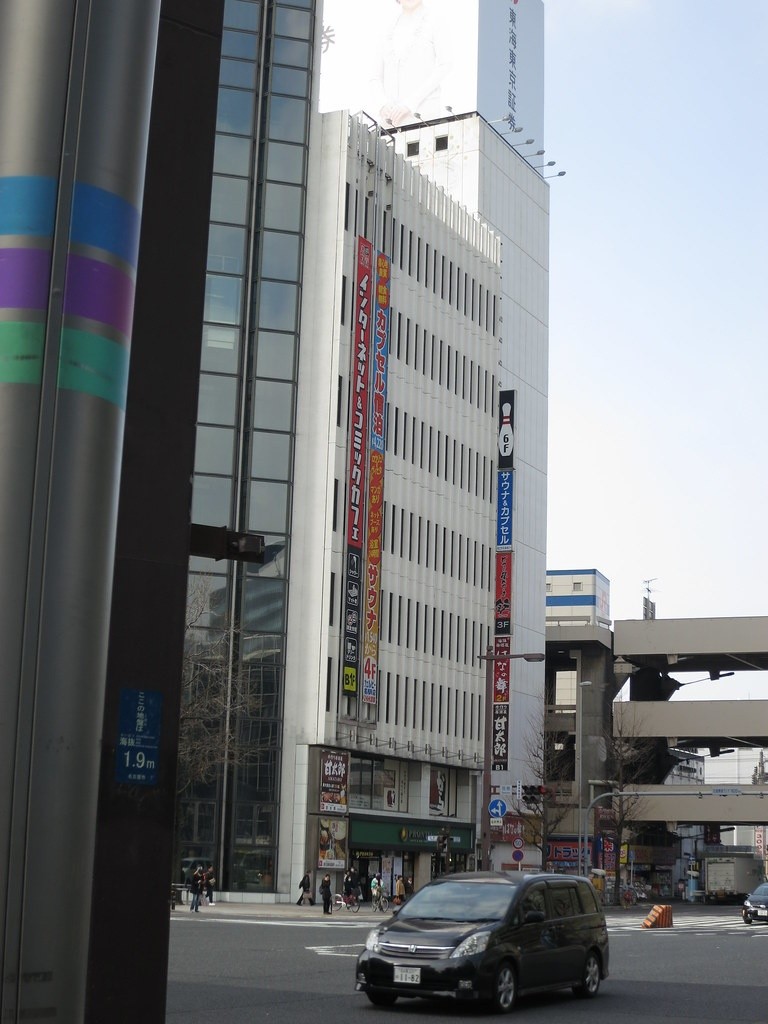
[522,785,552,795]
[522,793,540,803]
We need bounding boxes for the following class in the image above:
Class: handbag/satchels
[303,890,312,898]
[393,896,401,905]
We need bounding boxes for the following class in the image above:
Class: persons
[344,867,359,902]
[395,875,405,906]
[204,865,216,905]
[296,870,316,906]
[405,878,414,900]
[321,875,332,915]
[189,865,204,912]
[623,889,632,910]
[370,872,386,902]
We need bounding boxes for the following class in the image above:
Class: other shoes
[296,903,302,906]
[311,903,316,905]
[195,910,199,912]
[325,912,331,914]
[190,907,195,913]
[209,903,215,905]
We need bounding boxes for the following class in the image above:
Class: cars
[742,883,768,924]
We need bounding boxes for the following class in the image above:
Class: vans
[356,871,610,1016]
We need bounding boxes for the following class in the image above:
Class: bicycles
[372,886,389,912]
[330,888,361,913]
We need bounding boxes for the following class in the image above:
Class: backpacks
[319,880,324,894]
